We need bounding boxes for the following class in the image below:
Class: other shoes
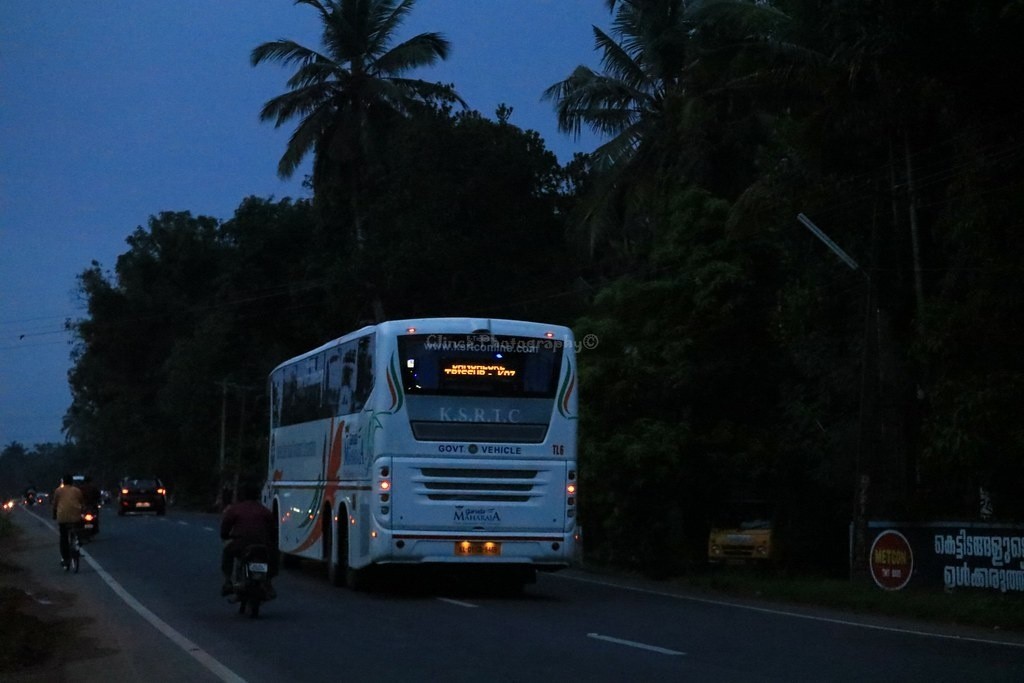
[61,559,70,566]
[222,583,232,596]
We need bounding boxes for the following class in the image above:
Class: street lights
[683,26,928,329]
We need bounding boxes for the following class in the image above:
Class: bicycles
[61,524,85,575]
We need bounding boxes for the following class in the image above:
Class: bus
[260,317,582,593]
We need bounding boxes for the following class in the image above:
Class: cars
[117,476,169,515]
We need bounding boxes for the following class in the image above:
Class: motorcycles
[222,534,277,615]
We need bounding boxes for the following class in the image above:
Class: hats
[239,484,259,500]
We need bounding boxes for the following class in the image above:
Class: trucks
[707,494,801,574]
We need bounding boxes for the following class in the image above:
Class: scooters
[81,504,101,542]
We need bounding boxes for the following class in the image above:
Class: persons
[53,474,101,566]
[221,483,279,600]
